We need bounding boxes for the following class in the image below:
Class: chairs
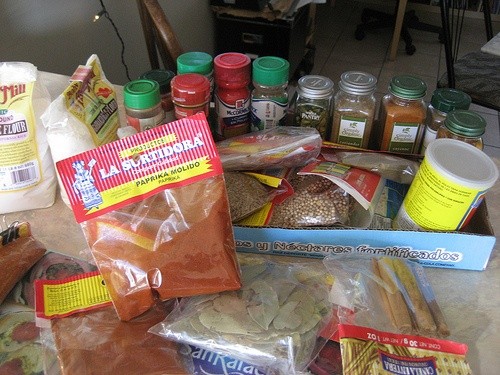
[437,0,500,122]
[136,0,186,71]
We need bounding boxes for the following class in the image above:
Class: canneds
[122,53,486,161]
[393,137,499,233]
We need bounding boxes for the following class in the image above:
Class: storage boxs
[233,142,497,273]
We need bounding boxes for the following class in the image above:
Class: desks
[0,71,500,375]
[212,6,314,82]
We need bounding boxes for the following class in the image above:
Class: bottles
[138,69,175,124]
[436,110,486,151]
[122,79,163,133]
[294,74,335,141]
[170,73,210,123]
[419,87,472,154]
[176,51,214,100]
[251,56,290,132]
[375,75,428,154]
[213,53,251,142]
[330,71,377,149]
